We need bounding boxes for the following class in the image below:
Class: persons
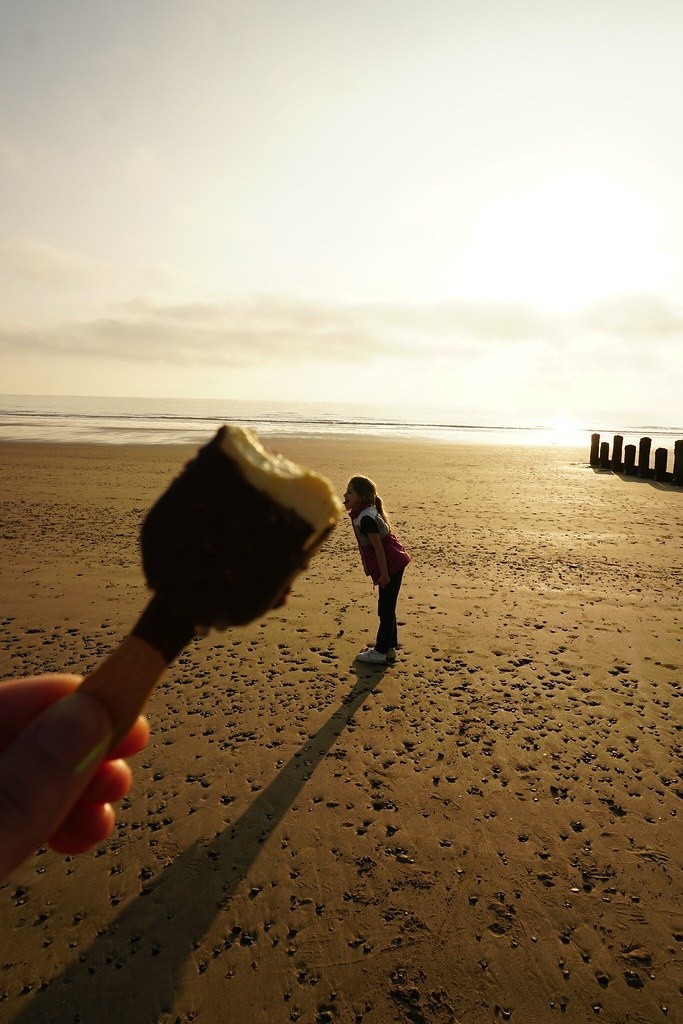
[1,674,148,876]
[343,476,411,662]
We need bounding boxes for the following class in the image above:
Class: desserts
[142,424,341,618]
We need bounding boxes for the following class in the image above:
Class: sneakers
[367,647,396,658]
[357,648,386,663]
